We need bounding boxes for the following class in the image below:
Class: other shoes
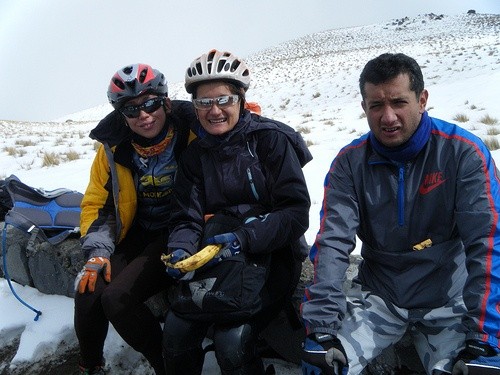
[77,356,106,375]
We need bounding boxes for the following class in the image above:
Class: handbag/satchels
[169,261,281,324]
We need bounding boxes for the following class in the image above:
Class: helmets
[107,63,168,112]
[184,48,250,94]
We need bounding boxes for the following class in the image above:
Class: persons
[73,64,202,375]
[301,53,500,375]
[158,49,311,375]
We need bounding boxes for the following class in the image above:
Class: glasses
[122,96,163,118]
[192,95,241,110]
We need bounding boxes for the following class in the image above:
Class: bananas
[161,244,223,272]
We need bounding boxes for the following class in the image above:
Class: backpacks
[0,173,84,320]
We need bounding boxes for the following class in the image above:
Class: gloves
[205,232,241,267]
[73,256,111,294]
[160,249,195,281]
[451,339,500,375]
[300,332,349,375]
